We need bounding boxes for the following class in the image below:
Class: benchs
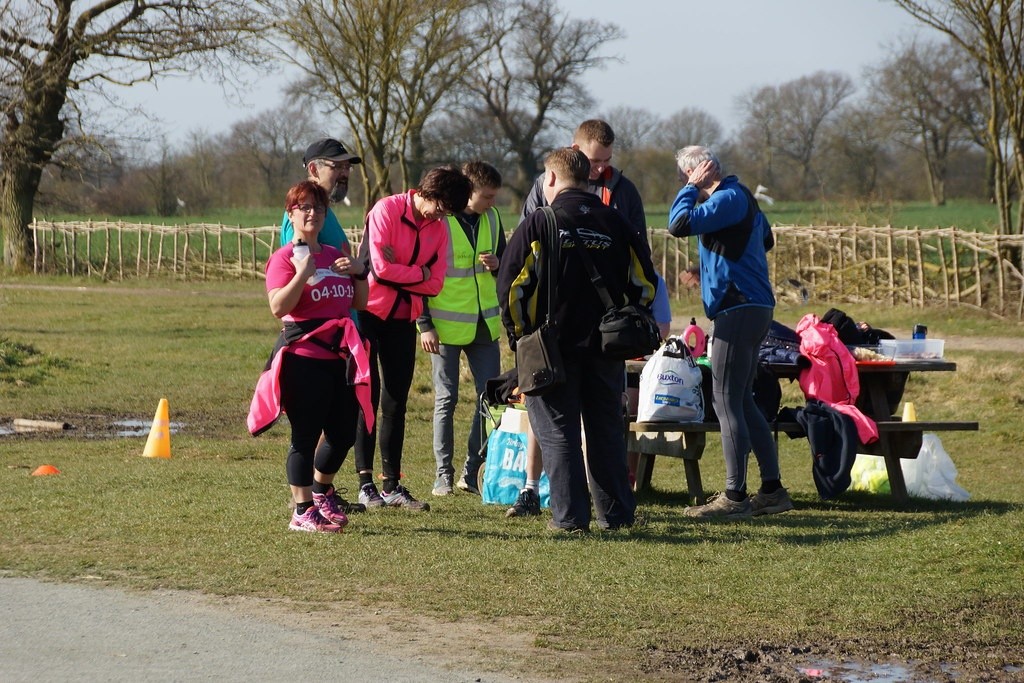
[626,414,980,510]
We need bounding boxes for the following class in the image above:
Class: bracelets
[354,261,371,280]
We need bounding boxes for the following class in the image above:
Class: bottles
[682,317,706,358]
[912,322,928,352]
[292,238,314,284]
[707,318,714,362]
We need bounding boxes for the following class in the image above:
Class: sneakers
[379,482,430,513]
[746,485,793,516]
[456,474,482,495]
[288,505,343,535]
[506,487,542,518]
[358,481,386,507]
[329,486,366,515]
[431,472,455,496]
[312,486,349,527]
[681,490,752,519]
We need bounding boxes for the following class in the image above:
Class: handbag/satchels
[598,303,662,361]
[636,335,706,422]
[483,429,551,508]
[516,319,567,397]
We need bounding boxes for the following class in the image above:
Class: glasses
[435,199,453,218]
[316,162,353,173]
[291,204,327,215]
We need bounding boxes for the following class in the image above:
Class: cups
[902,402,917,422]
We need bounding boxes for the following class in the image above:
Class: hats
[303,138,362,165]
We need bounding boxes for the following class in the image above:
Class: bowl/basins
[859,346,897,361]
[879,338,946,360]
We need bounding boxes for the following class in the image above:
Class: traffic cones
[901,401,917,422]
[142,397,171,459]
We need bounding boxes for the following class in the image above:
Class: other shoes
[594,514,628,529]
[546,519,591,534]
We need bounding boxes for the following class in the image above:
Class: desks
[625,354,955,511]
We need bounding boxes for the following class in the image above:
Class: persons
[517,120,671,485]
[668,145,793,523]
[278,139,367,515]
[505,422,544,517]
[416,161,507,496]
[266,180,369,537]
[354,166,475,510]
[496,146,660,537]
